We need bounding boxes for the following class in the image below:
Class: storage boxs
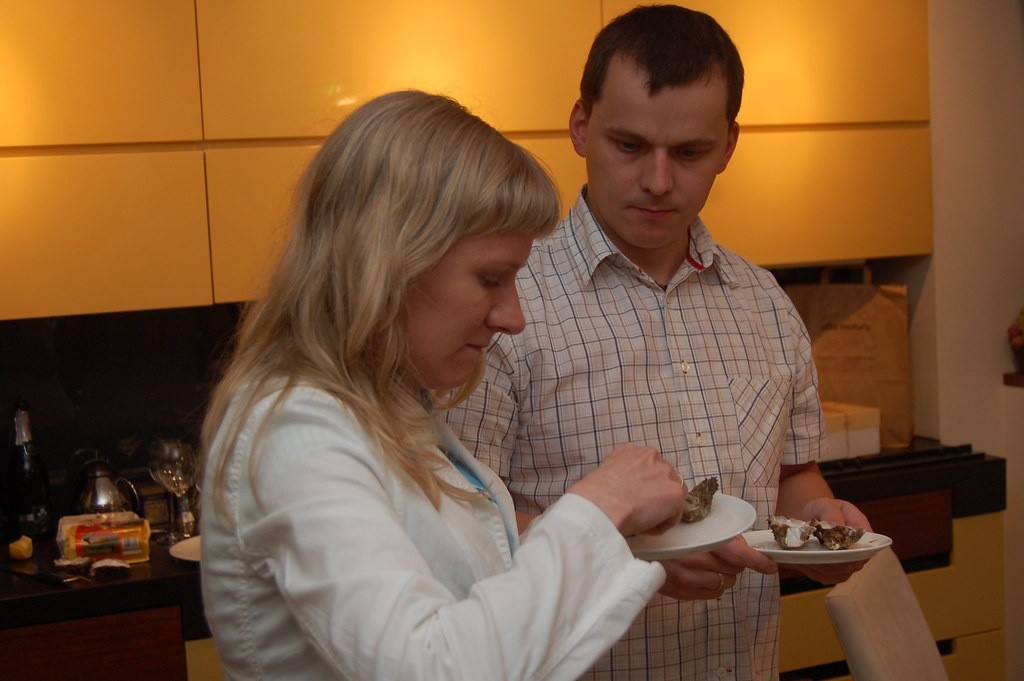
[821,401,883,461]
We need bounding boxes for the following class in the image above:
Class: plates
[169,535,201,562]
[741,530,892,564]
[624,492,757,560]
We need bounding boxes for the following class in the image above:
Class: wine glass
[148,439,181,546]
[155,442,199,541]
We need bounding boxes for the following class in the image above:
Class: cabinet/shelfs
[0,2,933,325]
[777,511,1006,680]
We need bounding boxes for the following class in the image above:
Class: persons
[196,88,688,681]
[422,4,873,681]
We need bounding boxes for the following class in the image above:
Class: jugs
[82,459,139,514]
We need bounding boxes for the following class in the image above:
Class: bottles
[7,407,50,544]
[176,494,195,540]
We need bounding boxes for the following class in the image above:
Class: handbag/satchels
[783,263,914,450]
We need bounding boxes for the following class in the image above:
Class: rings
[676,472,684,487]
[712,572,725,598]
